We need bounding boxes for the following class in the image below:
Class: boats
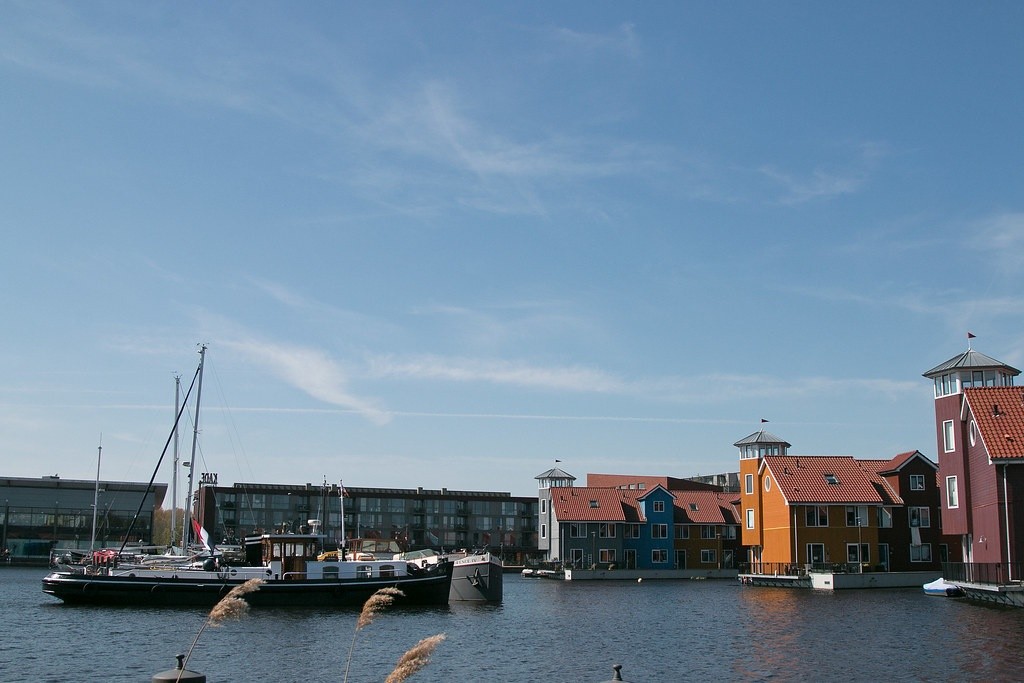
[392,523,504,608]
[922,577,967,598]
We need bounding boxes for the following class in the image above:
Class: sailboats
[40,339,456,610]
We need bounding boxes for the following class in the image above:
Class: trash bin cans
[862,566,871,572]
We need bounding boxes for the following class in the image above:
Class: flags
[192,518,217,555]
[342,485,350,498]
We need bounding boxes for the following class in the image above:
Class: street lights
[856,517,862,574]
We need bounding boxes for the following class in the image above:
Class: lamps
[976,535,987,544]
